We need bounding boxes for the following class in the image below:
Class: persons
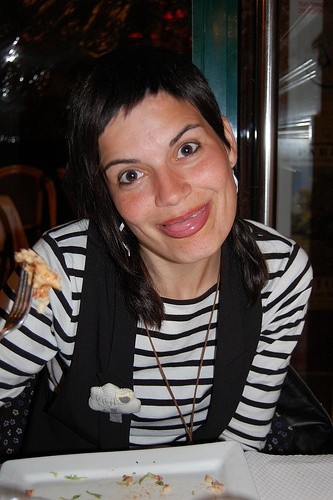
[0,45,314,454]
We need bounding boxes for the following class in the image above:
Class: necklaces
[137,266,221,442]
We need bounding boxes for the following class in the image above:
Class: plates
[0,440,259,500]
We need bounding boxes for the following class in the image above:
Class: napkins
[245,451,333,500]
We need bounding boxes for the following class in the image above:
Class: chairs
[0,165,56,246]
[0,194,30,288]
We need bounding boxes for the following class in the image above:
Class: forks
[0,267,33,340]
